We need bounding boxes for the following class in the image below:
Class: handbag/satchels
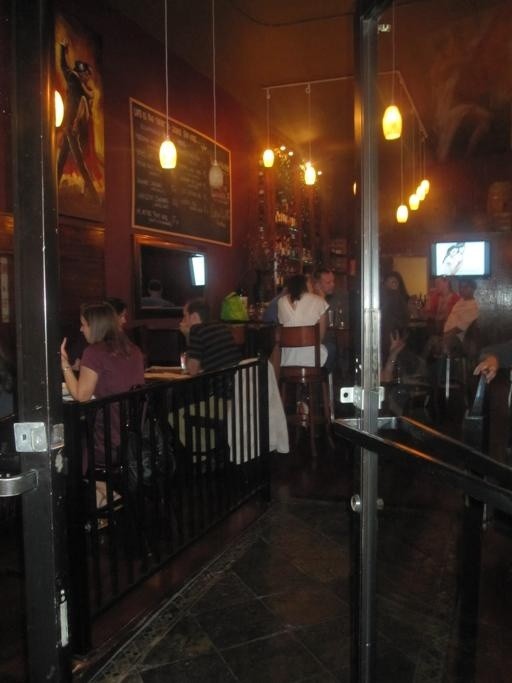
[220,292,249,321]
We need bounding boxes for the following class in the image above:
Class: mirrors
[132,232,208,318]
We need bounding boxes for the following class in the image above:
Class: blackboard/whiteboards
[129,98,233,247]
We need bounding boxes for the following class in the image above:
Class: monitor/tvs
[189,255,205,286]
[428,240,490,279]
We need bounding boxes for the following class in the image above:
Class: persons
[335,271,512,467]
[56,36,102,205]
[165,300,241,479]
[456,241,476,275]
[313,269,340,311]
[441,241,466,275]
[72,298,128,374]
[143,277,183,319]
[59,299,147,534]
[265,273,328,425]
[259,275,293,381]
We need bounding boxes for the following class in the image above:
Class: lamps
[209,0,223,189]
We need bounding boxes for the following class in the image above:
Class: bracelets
[61,365,73,373]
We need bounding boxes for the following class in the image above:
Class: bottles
[438,355,467,405]
[255,181,312,288]
[239,285,268,323]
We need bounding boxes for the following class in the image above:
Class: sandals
[84,487,126,532]
